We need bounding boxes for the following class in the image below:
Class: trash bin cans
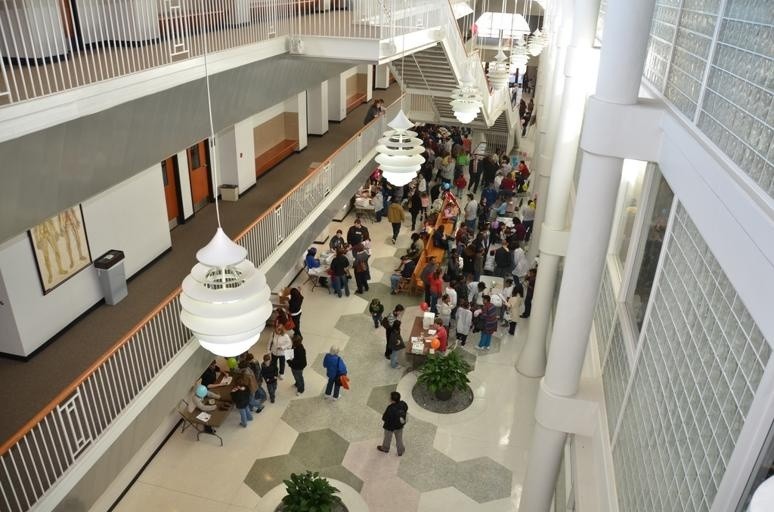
[220,184,238,201]
[94,249,128,306]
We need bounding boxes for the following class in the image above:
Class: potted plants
[418,351,474,401]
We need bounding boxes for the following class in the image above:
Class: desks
[193,372,244,445]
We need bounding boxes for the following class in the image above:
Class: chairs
[177,399,201,433]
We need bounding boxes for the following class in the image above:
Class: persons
[376,391,408,457]
[191,72,537,434]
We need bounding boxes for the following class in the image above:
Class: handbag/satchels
[285,320,296,329]
[335,374,345,386]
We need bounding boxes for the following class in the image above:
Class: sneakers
[324,393,341,400]
[256,405,264,413]
[279,375,284,380]
[377,446,389,452]
[398,448,405,456]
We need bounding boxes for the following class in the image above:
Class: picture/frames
[27,203,93,295]
